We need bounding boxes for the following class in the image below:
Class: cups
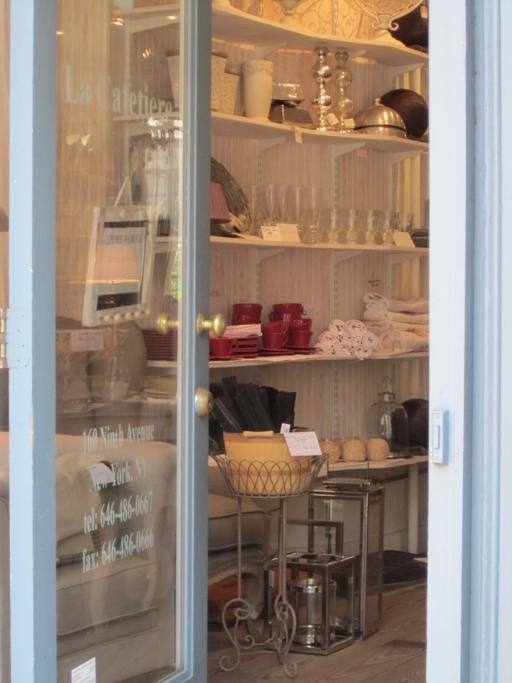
[241,58,274,120]
[209,300,312,357]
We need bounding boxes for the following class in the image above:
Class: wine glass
[248,182,414,245]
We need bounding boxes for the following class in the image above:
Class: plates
[233,337,320,358]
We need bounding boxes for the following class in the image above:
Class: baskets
[209,449,329,498]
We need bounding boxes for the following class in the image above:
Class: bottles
[367,374,414,461]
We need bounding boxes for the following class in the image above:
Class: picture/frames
[79,203,159,329]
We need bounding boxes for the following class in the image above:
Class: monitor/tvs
[55,204,157,328]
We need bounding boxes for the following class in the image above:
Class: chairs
[0,431,176,683]
[57,320,260,626]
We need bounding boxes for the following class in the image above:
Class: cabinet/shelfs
[111,0,430,557]
